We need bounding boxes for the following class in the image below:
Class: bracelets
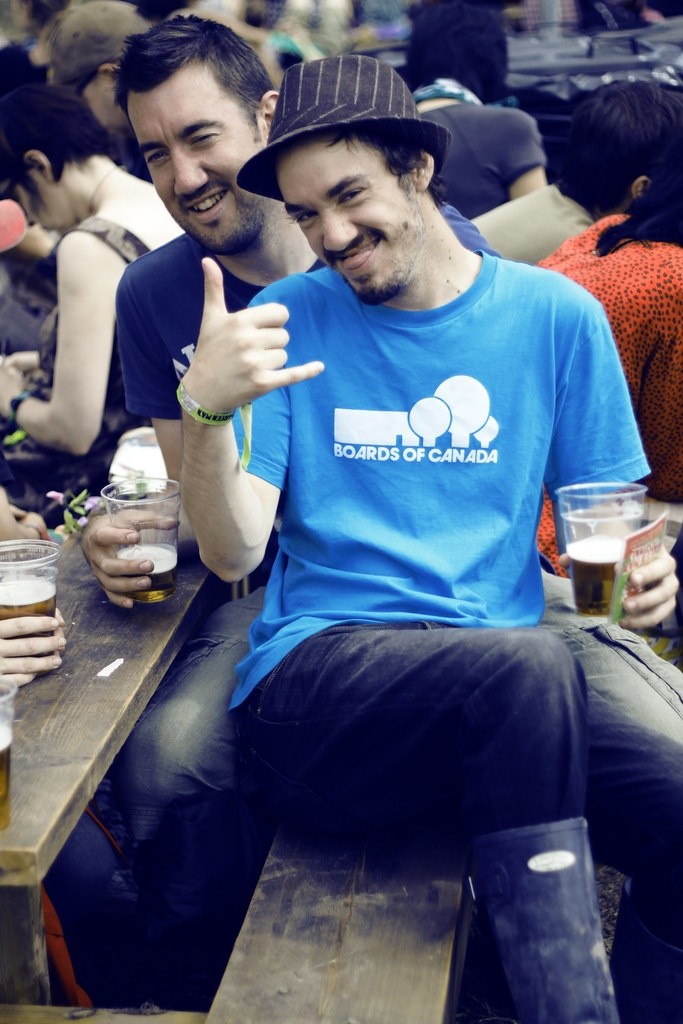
[176,380,236,425]
[7,390,32,426]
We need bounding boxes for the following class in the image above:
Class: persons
[0,0,683,691]
[180,54,680,1024]
[82,14,683,1024]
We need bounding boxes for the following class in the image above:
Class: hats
[237,55,452,202]
[45,0,152,85]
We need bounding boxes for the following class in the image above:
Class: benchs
[203,825,470,1024]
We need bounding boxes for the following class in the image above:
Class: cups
[554,482,649,617]
[0,680,18,831]
[99,478,181,603]
[0,539,63,675]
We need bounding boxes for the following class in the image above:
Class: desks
[0,495,222,1003]
